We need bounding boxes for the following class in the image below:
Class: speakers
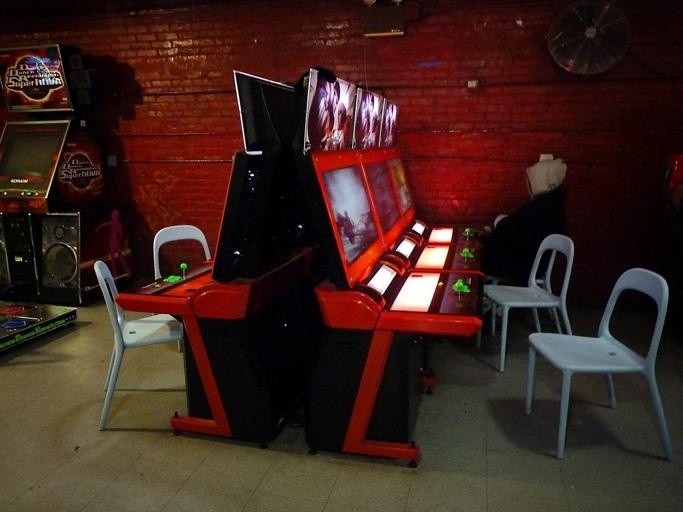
[0,214,40,296]
[41,207,138,306]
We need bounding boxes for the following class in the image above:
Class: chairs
[95,225,211,430]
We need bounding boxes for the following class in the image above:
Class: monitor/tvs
[305,146,413,265]
[211,151,299,282]
[0,135,60,177]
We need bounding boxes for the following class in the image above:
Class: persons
[474,155,569,341]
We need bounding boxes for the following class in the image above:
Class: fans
[546,2,633,76]
